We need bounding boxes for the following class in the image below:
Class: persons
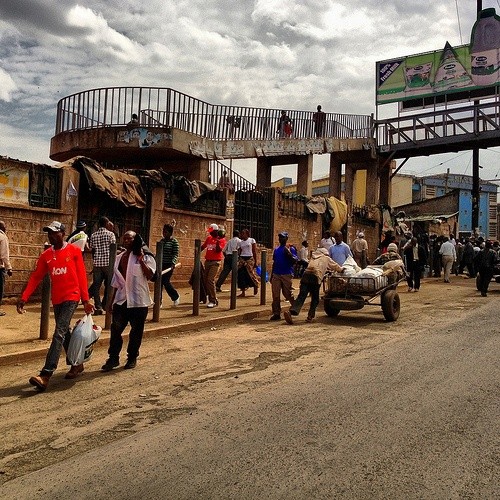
[320,230,336,250]
[351,232,368,263]
[314,105,326,137]
[216,230,241,292]
[237,228,260,298]
[270,232,295,320]
[66,220,90,255]
[0,221,12,316]
[285,248,343,325]
[328,231,353,266]
[300,240,310,268]
[202,224,226,308]
[159,224,180,309]
[381,231,500,297]
[102,231,156,370]
[144,132,154,146]
[280,111,291,136]
[106,221,118,240]
[17,220,97,392]
[132,131,141,138]
[373,243,401,265]
[127,114,138,126]
[88,216,116,316]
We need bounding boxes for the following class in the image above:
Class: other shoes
[102,355,119,369]
[305,316,314,322]
[64,363,85,379]
[415,288,420,292]
[408,286,412,292]
[237,293,245,297]
[254,287,257,295]
[283,310,294,325]
[207,302,217,308]
[270,315,281,321]
[30,374,50,391]
[174,293,180,306]
[124,358,136,368]
[216,287,221,292]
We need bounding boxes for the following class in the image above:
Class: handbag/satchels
[256,266,269,282]
[67,312,102,366]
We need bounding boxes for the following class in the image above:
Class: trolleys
[320,263,410,322]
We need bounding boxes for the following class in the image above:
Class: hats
[387,243,398,253]
[358,232,365,238]
[316,247,330,256]
[207,224,219,233]
[278,232,289,237]
[43,221,65,232]
